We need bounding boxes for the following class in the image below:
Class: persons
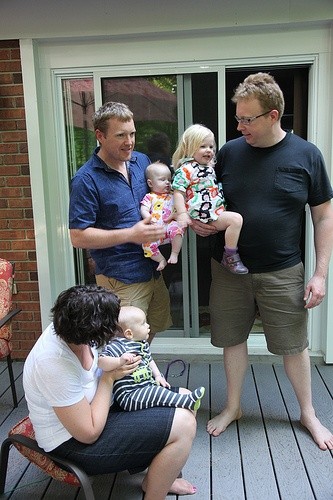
[70,102,174,347]
[190,72,333,451]
[140,159,188,272]
[171,124,249,275]
[22,285,196,500]
[98,306,205,411]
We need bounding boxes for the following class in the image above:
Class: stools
[0,416,182,500]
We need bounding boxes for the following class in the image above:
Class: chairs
[0,257,22,408]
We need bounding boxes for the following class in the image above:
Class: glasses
[234,110,272,126]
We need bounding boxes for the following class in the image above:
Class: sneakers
[220,251,249,275]
